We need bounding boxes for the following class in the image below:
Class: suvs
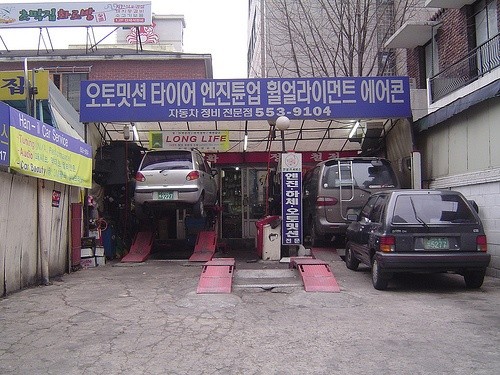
[301,156,401,248]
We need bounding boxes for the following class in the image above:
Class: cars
[344,187,492,291]
[134,147,219,222]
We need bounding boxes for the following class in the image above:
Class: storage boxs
[81,248,94,258]
[88,229,102,239]
[97,257,105,265]
[81,257,96,268]
[94,246,104,256]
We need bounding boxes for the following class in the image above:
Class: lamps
[348,119,361,140]
[244,132,248,152]
[132,123,140,142]
[277,116,290,130]
